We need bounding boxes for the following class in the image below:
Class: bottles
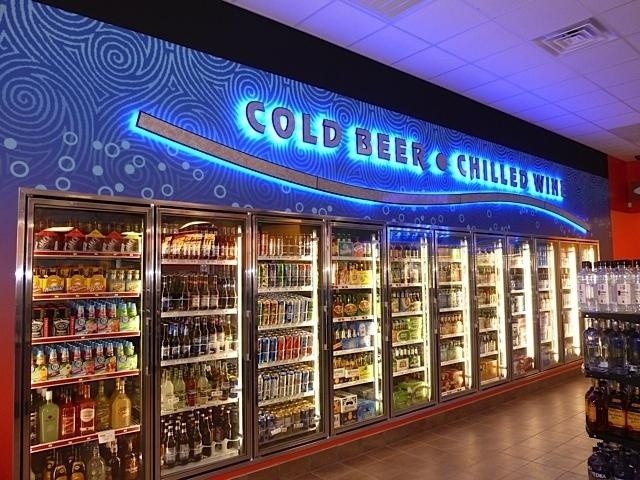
[538,243,595,369]
[252,227,321,440]
[476,246,501,382]
[25,215,141,480]
[510,244,536,375]
[587,443,640,480]
[585,379,640,437]
[576,256,640,316]
[159,213,235,467]
[581,316,639,378]
[433,244,467,400]
[390,241,432,413]
[329,231,378,430]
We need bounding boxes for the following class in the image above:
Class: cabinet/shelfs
[580,310,640,450]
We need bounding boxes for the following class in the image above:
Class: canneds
[257,261,311,286]
[260,231,313,255]
[257,293,313,326]
[258,364,315,444]
[257,329,313,363]
